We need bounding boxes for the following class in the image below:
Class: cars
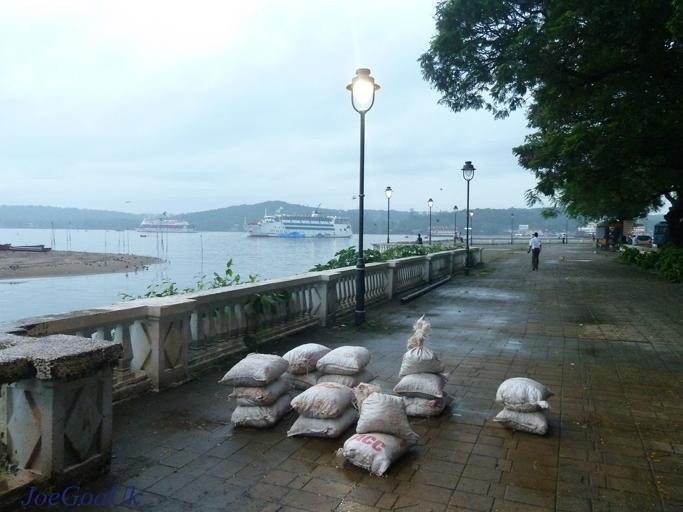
[635,234,652,248]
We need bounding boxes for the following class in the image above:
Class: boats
[10,244,50,253]
[241,206,352,239]
[431,225,459,237]
[133,215,199,234]
[0,242,12,251]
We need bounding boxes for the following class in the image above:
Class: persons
[527,233,540,271]
[562,233,565,243]
[416,233,422,243]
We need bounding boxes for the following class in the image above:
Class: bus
[652,221,668,248]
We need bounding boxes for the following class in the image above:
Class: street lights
[345,67,380,326]
[565,215,570,244]
[384,185,393,243]
[511,212,514,245]
[427,198,434,245]
[453,205,457,246]
[459,160,476,270]
[469,210,474,246]
